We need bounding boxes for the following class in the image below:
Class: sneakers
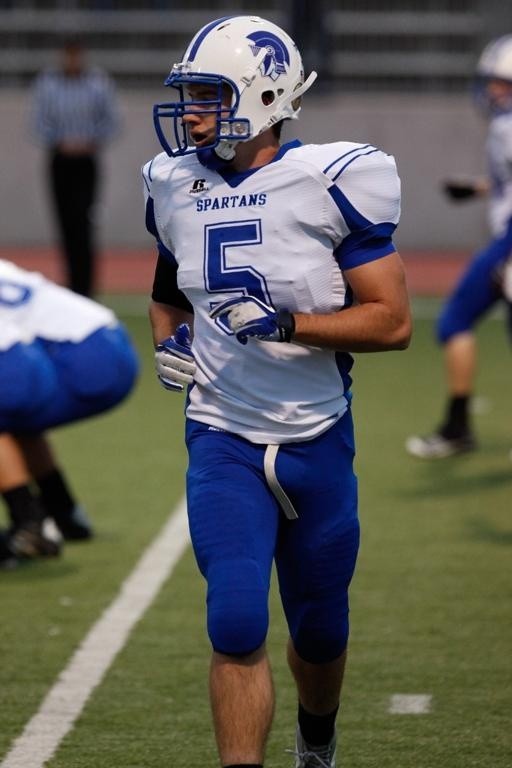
[406,428,470,457]
[285,721,337,767]
[3,507,90,560]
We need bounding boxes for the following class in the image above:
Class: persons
[141,14,413,767]
[404,32,510,460]
[29,36,131,294]
[0,259,139,559]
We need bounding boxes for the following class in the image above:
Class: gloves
[155,324,196,393]
[208,296,295,345]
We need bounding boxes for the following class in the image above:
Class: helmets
[153,14,319,169]
[477,36,511,112]
